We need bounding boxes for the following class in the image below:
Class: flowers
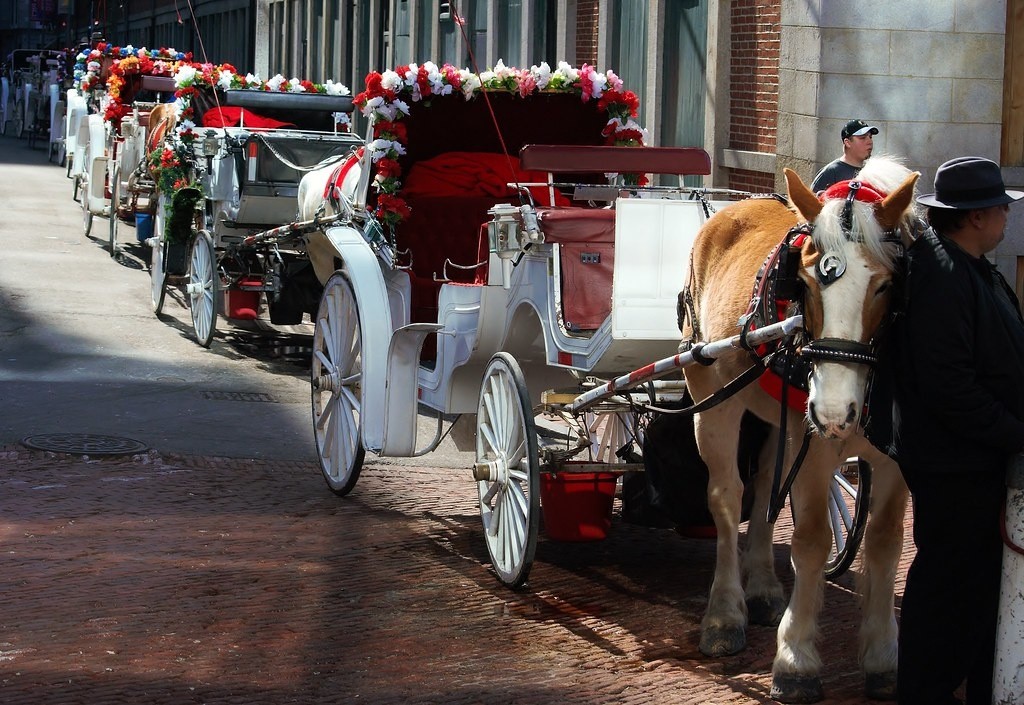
[27,43,648,257]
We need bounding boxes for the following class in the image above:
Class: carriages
[291,87,918,705]
[0,41,368,354]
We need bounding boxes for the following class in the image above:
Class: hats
[841,119,879,143]
[915,157,1024,209]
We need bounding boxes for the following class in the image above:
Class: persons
[810,119,879,194]
[866,155,1024,705]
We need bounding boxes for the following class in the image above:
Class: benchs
[21,59,712,329]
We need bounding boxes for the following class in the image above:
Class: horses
[676,151,919,698]
[294,150,364,279]
[146,101,180,158]
